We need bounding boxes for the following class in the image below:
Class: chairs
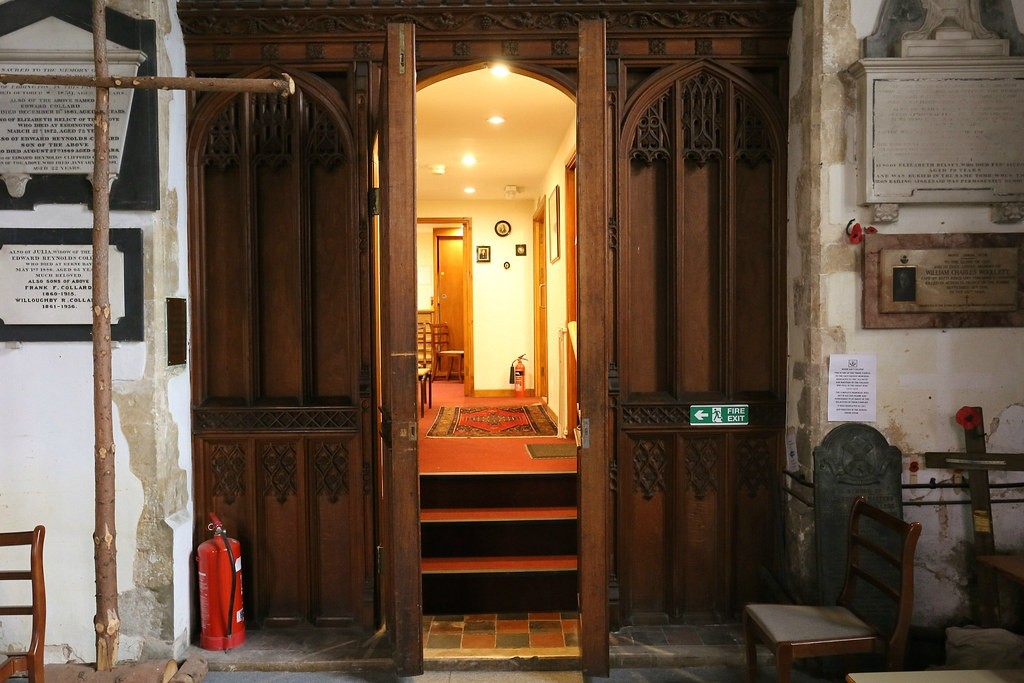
[744,496,921,683]
[417,323,464,419]
[0,524,46,683]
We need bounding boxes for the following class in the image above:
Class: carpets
[426,404,558,438]
[525,443,577,459]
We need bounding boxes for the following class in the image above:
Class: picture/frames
[516,244,526,256]
[477,246,490,262]
[495,221,512,237]
[549,185,560,264]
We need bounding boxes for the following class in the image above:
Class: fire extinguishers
[510,353,529,398]
[195,511,247,653]
